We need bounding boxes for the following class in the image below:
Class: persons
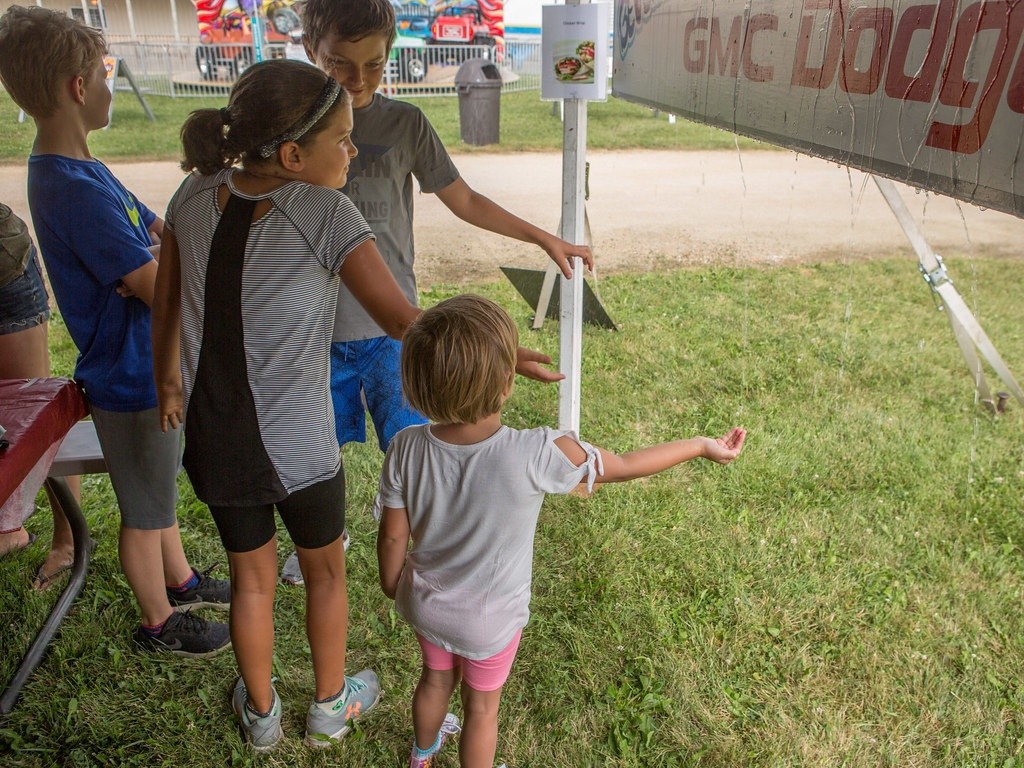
[375,294,749,768]
[1,6,229,660]
[283,0,595,585]
[0,203,82,590]
[149,60,570,747]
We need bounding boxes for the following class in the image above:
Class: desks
[0,376,90,717]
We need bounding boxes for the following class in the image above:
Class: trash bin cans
[454,57,503,145]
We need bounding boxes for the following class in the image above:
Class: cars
[386,0,501,82]
[194,1,307,83]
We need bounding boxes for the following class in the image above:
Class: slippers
[34,536,74,592]
[0,533,36,563]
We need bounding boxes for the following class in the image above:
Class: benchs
[46,422,107,477]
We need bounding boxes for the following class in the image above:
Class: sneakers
[133,605,232,658]
[410,713,461,768]
[281,528,350,585]
[304,670,381,749]
[232,676,284,754]
[166,562,231,613]
